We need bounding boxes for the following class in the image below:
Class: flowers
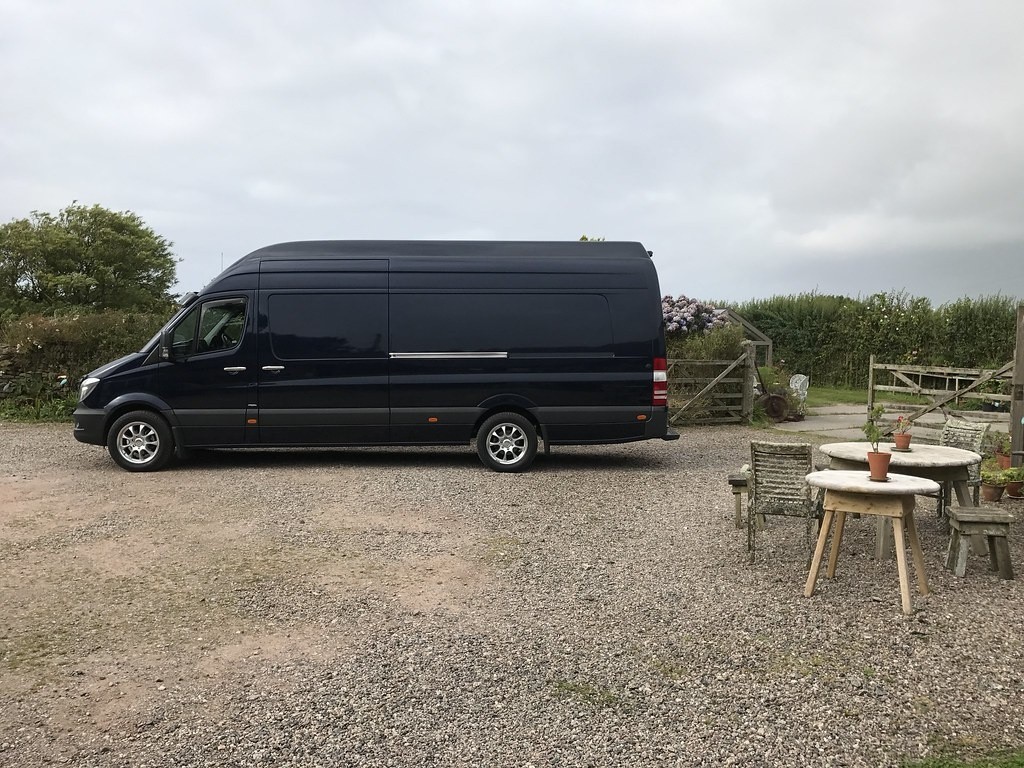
[894,416,914,434]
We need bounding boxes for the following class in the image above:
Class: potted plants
[1004,466,1024,497]
[980,470,1008,501]
[993,429,1010,469]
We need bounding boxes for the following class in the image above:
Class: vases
[860,404,892,478]
[894,432,912,449]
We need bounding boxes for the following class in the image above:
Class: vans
[71,239,682,473]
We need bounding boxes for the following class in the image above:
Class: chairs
[915,418,992,517]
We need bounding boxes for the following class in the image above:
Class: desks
[819,442,988,558]
[803,470,941,614]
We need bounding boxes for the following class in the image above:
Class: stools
[728,474,767,529]
[944,506,1014,580]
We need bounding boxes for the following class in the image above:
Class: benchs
[740,438,823,568]
